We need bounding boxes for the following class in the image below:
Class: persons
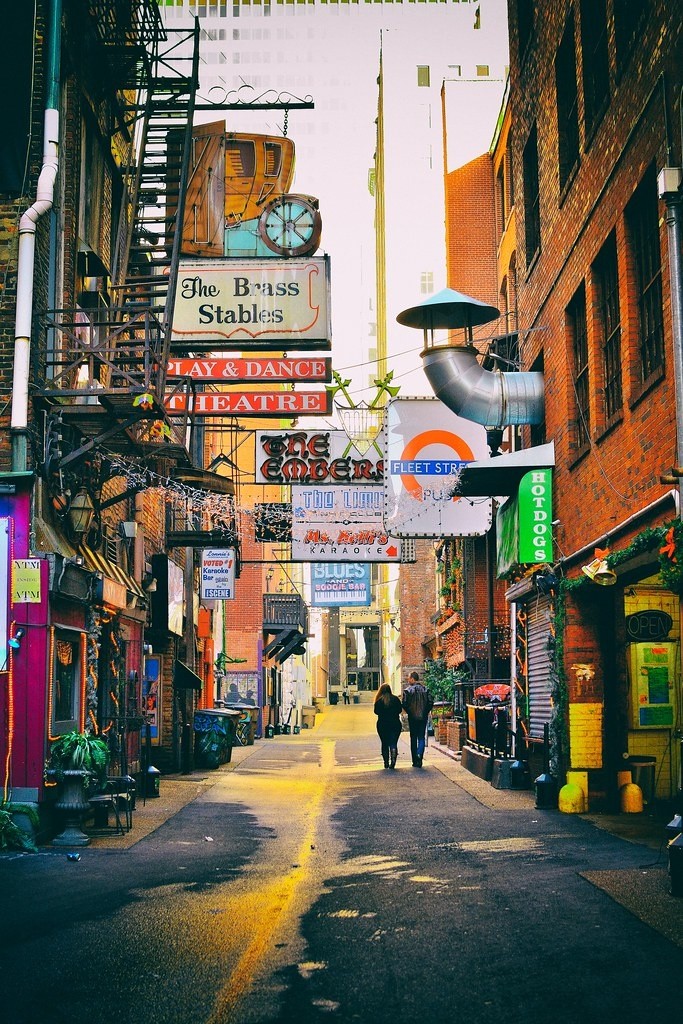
[343,686,346,704]
[346,686,351,704]
[374,683,402,768]
[402,672,434,767]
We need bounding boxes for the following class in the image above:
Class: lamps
[422,656,434,673]
[61,556,87,568]
[49,482,98,545]
[8,627,25,649]
[85,569,105,585]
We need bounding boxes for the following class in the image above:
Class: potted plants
[45,730,111,847]
[439,586,451,601]
[445,573,456,591]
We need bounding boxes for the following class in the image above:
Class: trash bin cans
[329,692,338,705]
[195,708,240,769]
[222,702,260,747]
[353,696,358,703]
[629,756,657,808]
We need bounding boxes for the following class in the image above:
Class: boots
[389,749,399,768]
[381,754,389,768]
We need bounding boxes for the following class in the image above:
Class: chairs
[81,779,125,837]
[107,774,137,829]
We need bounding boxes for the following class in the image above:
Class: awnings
[449,440,555,498]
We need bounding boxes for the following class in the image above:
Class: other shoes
[417,753,422,768]
[412,763,416,767]
